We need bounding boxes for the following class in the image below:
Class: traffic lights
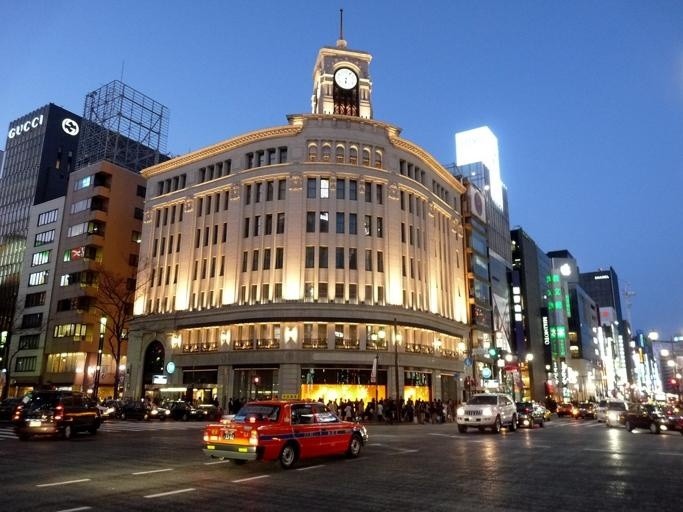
[488,348,498,360]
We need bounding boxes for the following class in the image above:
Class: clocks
[334,67,359,91]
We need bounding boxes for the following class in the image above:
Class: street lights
[371,330,386,414]
[93,313,107,395]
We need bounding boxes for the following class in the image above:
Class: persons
[299,396,457,425]
[101,396,277,424]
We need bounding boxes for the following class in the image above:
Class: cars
[202,399,369,471]
[96,399,220,421]
[556,398,682,434]
[11,391,101,441]
[456,393,551,434]
